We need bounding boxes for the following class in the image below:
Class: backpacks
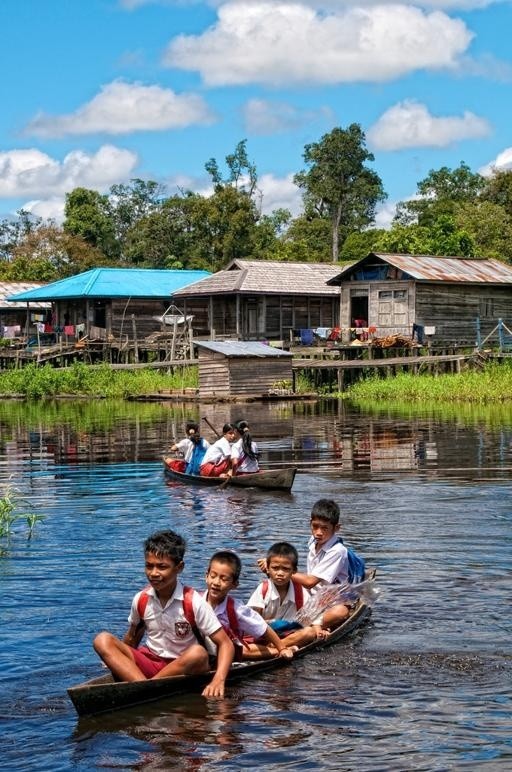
[255,619,304,642]
[184,437,207,476]
[332,536,366,612]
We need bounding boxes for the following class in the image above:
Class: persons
[195,550,300,659]
[256,498,366,630]
[164,419,211,474]
[199,422,232,479]
[92,529,236,698]
[219,420,260,479]
[241,541,330,659]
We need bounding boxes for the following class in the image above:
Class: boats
[163,457,297,491]
[67,602,370,717]
